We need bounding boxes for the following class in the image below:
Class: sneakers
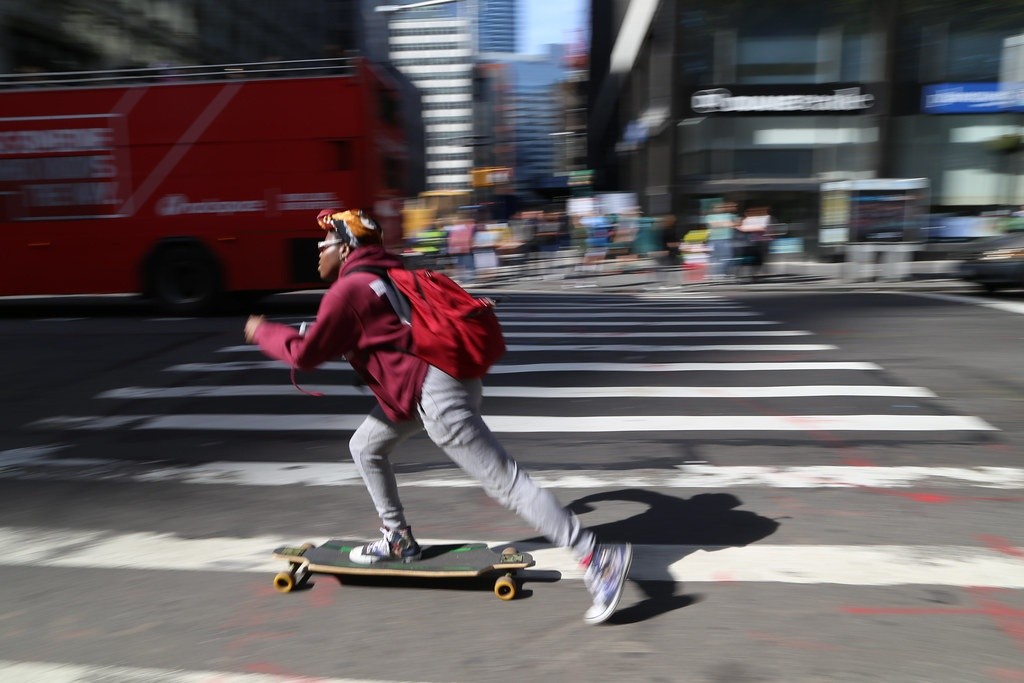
[349,524,421,565]
[584,542,634,625]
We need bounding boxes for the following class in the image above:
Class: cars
[960,231,1023,293]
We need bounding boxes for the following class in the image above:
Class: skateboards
[269,540,534,600]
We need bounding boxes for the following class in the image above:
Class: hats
[324,208,383,250]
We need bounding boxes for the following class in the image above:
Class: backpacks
[389,268,505,381]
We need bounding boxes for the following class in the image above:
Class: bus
[2,0,427,320]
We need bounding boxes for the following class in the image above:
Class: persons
[243,205,634,625]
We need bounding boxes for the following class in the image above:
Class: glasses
[317,239,342,248]
